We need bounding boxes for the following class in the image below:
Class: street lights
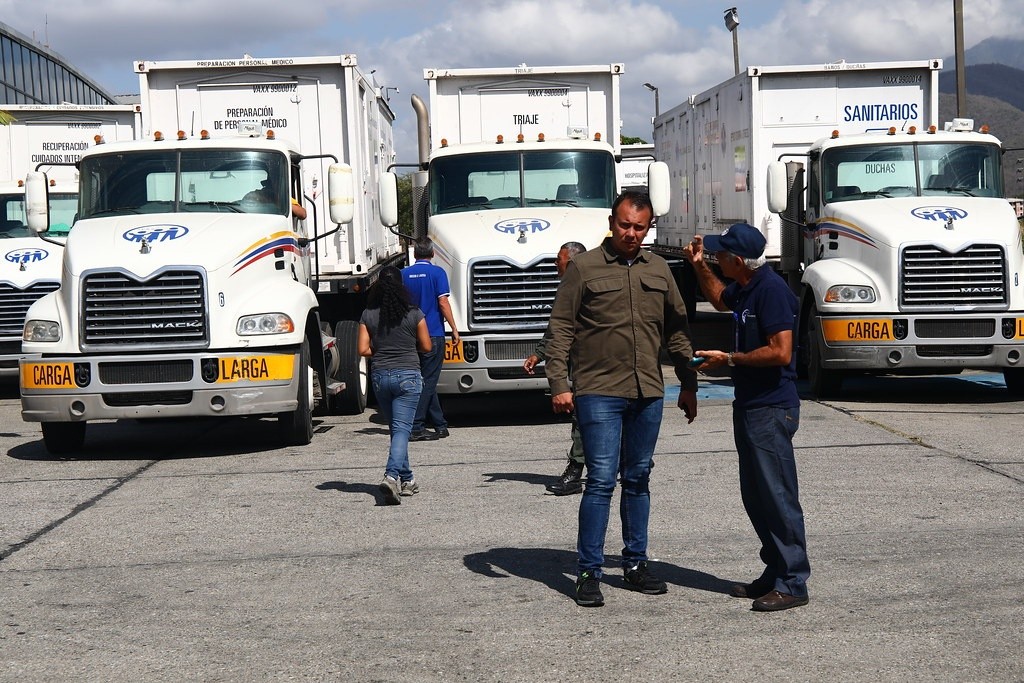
[721,7,741,75]
[642,83,660,118]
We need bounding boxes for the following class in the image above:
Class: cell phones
[689,356,706,367]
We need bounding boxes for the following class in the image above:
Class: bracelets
[728,352,736,367]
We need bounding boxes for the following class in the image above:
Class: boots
[546,459,584,495]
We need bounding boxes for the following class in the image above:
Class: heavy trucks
[376,62,673,396]
[0,53,409,452]
[652,59,1023,403]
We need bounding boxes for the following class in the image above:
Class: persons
[523,242,656,495]
[545,191,699,606]
[683,222,811,612]
[241,164,306,221]
[399,237,460,443]
[359,266,432,505]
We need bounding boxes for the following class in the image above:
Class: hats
[703,223,767,258]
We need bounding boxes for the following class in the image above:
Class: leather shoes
[753,591,809,610]
[733,578,774,598]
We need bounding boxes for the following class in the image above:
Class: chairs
[0,219,23,236]
[928,175,965,190]
[557,183,598,201]
[833,186,861,198]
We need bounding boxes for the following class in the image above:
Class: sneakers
[409,430,439,440]
[400,478,419,495]
[623,561,667,594]
[575,569,605,605]
[379,475,401,505]
[435,429,449,438]
